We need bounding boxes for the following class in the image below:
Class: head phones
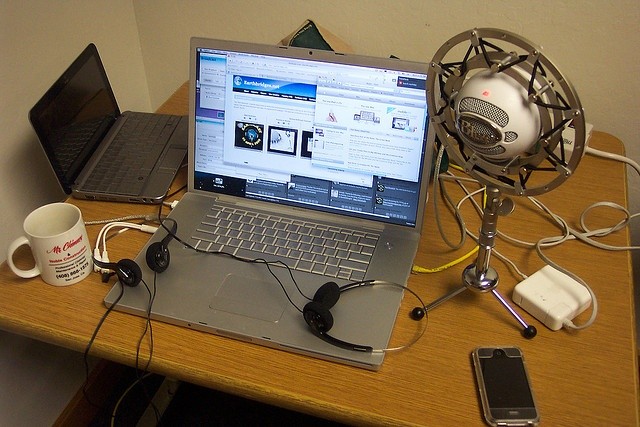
[93,216,181,283]
[302,280,429,353]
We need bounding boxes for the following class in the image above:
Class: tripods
[409,196,538,339]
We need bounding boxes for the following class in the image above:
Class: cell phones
[471,345,538,426]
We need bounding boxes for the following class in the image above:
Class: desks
[0,76,638,425]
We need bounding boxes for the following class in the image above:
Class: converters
[513,265,593,332]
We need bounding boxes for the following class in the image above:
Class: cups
[7,203,94,287]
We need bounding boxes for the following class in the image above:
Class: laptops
[102,37,439,368]
[27,43,191,203]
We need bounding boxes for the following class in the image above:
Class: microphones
[422,29,584,195]
